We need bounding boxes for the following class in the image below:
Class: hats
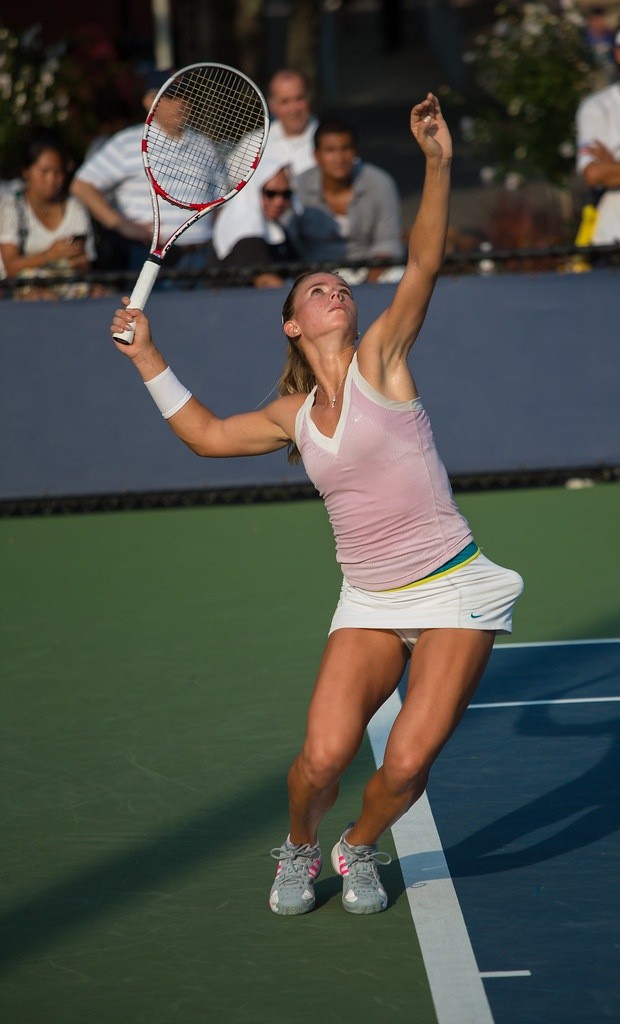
[141,62,195,98]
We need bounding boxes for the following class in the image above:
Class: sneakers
[265,834,326,914]
[328,823,392,916]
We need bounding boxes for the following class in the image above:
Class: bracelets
[143,366,192,419]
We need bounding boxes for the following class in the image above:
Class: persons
[0,0,620,302]
[110,93,524,914]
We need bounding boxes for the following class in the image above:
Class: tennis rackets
[115,59,274,346]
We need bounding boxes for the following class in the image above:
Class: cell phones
[68,233,87,245]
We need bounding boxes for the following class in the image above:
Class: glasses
[263,187,294,200]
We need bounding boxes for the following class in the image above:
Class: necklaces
[316,371,346,411]
